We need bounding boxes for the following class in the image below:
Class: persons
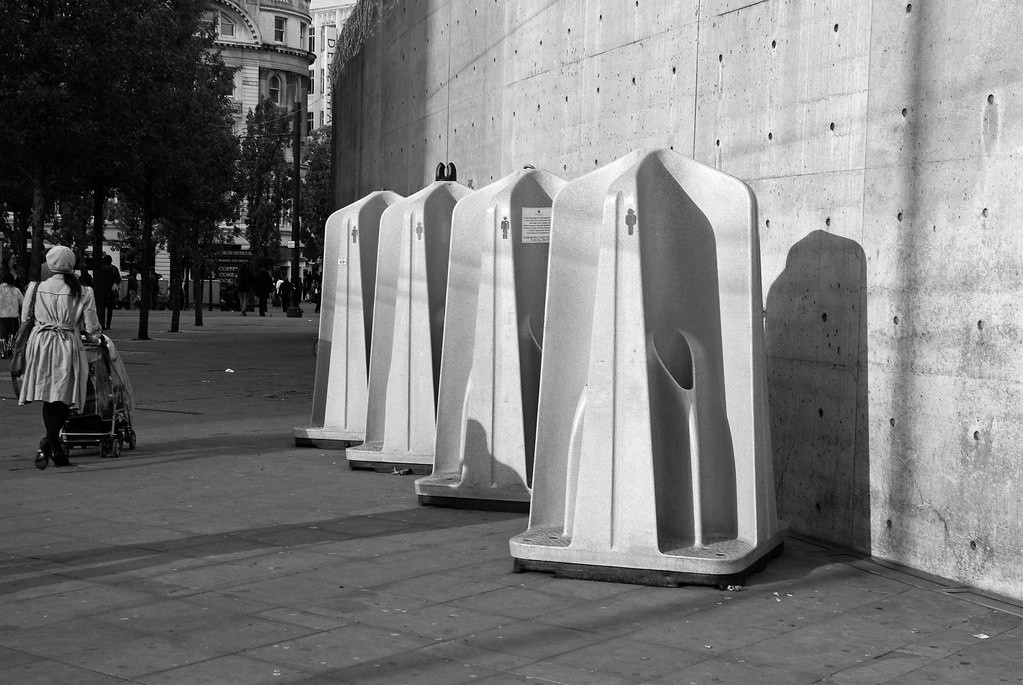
[0,273,24,357]
[18,245,101,470]
[237,261,322,317]
[79,254,162,330]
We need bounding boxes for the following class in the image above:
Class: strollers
[51,330,138,458]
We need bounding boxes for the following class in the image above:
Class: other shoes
[241,310,246,316]
[1,352,7,359]
[260,312,265,316]
[35,437,50,469]
[50,446,70,466]
[7,347,14,357]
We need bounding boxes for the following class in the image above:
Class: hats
[46,245,76,274]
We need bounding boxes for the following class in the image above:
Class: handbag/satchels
[10,279,41,377]
[112,284,120,299]
